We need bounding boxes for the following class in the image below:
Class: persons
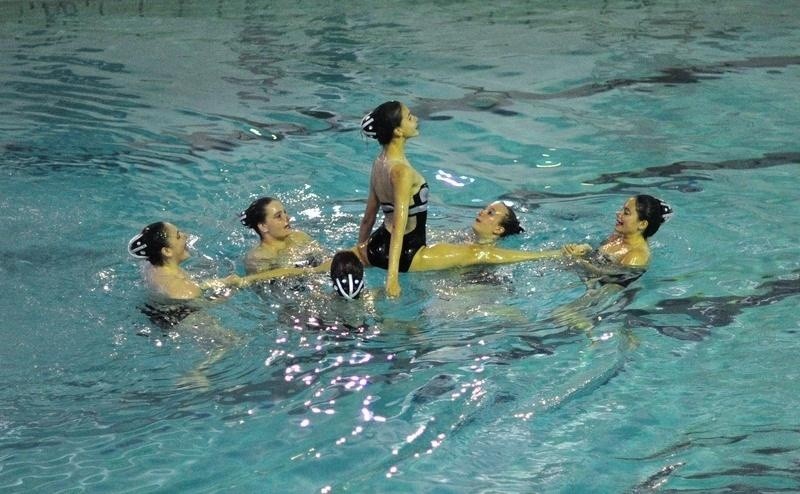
[202,99,592,291]
[239,196,329,325]
[552,195,673,344]
[431,200,525,323]
[129,222,239,389]
[328,252,428,346]
